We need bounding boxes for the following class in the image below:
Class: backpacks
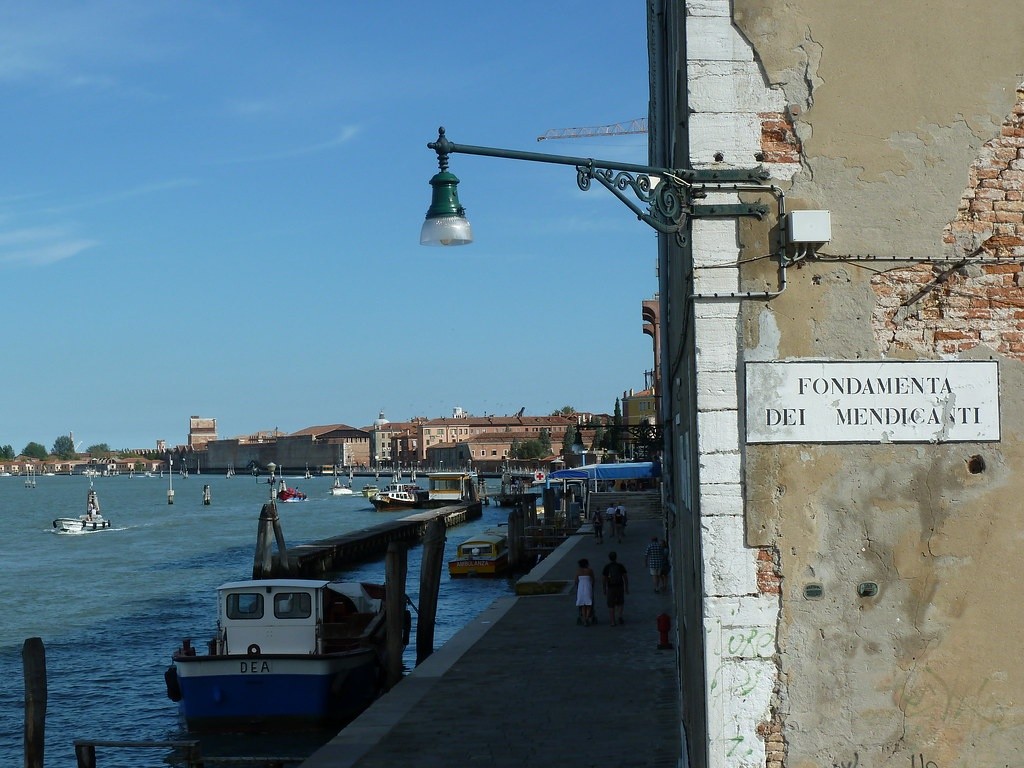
[593,511,602,527]
[608,563,621,585]
[616,514,622,524]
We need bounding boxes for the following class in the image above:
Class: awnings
[546,461,662,480]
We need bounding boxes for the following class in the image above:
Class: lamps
[572,423,664,455]
[418,125,770,247]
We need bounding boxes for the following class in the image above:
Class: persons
[605,503,615,538]
[600,482,606,492]
[88,500,93,521]
[602,552,629,627]
[575,559,595,626]
[591,506,603,544]
[614,509,623,544]
[620,479,632,492]
[614,502,627,536]
[512,477,525,494]
[645,536,669,594]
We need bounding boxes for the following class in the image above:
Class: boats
[165,579,412,734]
[330,487,353,495]
[369,471,474,512]
[448,471,609,578]
[278,486,308,502]
[52,475,111,533]
[361,484,381,499]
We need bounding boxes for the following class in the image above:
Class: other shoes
[661,587,666,592]
[618,616,624,624]
[584,620,589,627]
[654,588,659,594]
[611,624,616,627]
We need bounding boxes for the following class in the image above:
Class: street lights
[440,461,443,472]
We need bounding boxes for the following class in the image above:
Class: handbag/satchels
[660,561,671,576]
[606,514,613,520]
